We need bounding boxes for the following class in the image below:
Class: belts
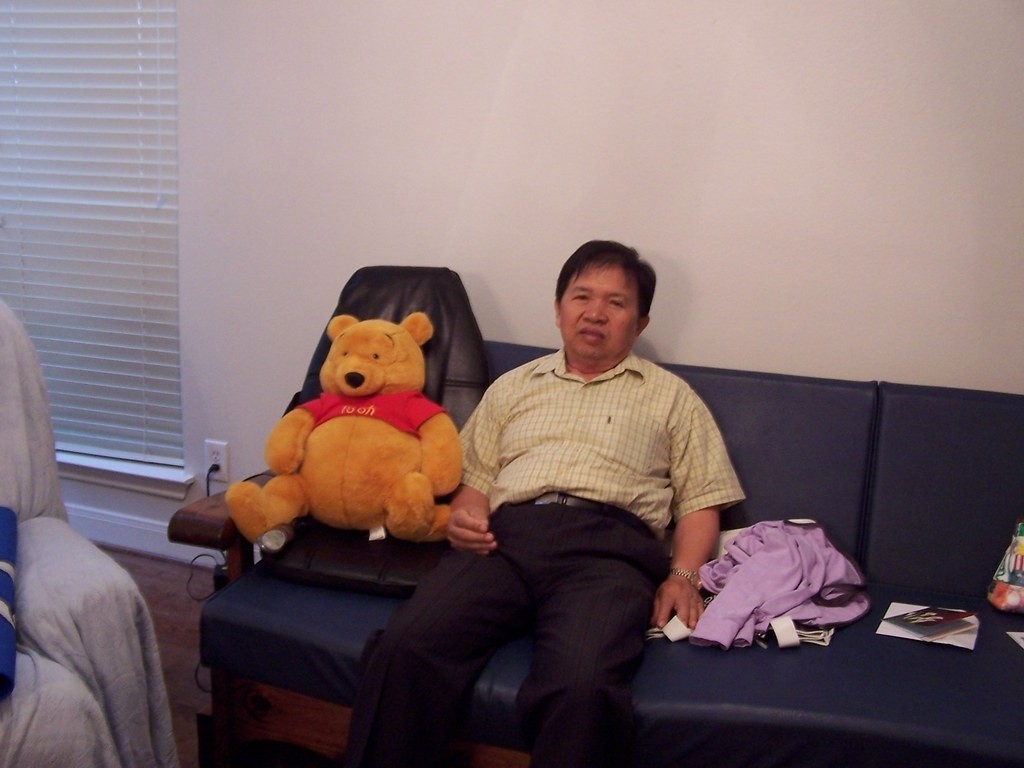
[525,493,656,541]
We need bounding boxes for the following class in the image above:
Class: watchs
[671,568,703,591]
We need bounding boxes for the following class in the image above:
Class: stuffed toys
[225,312,463,542]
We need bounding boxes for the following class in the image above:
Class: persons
[346,240,746,768]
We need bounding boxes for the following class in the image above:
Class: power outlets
[204,438,229,483]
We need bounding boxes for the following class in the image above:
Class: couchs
[208,335,1024,768]
[0,302,181,768]
